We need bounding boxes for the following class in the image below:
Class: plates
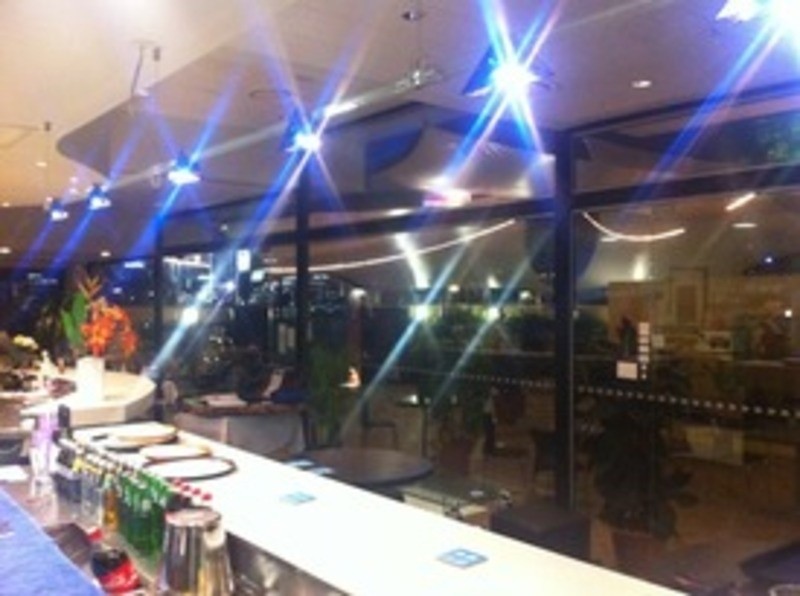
[140,457,235,481]
[98,424,178,453]
[137,442,211,462]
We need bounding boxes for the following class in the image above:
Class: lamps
[50,152,203,221]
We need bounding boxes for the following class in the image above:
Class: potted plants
[587,361,697,568]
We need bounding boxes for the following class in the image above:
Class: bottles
[26,410,220,596]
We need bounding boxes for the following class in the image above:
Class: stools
[495,498,589,562]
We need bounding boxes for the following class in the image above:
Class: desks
[285,447,439,499]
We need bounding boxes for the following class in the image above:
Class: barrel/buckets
[154,505,235,596]
